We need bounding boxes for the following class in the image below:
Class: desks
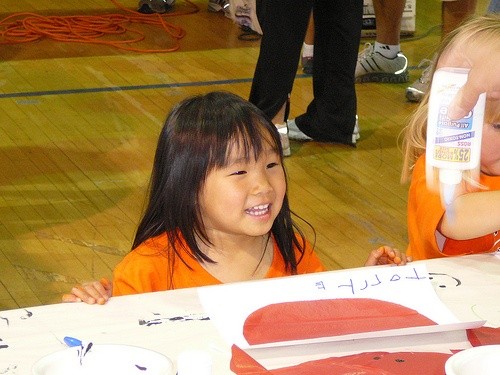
[0,251,500,375]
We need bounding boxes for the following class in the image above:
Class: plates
[444,344,500,375]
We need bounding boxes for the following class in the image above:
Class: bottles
[424,65,487,209]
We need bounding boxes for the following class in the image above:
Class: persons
[247,0,500,158]
[397,12,500,261]
[62,91,412,305]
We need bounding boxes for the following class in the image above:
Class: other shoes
[275,121,291,156]
[301,56,314,74]
[287,114,360,144]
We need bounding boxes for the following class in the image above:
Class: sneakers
[406,52,439,102]
[137,0,176,14]
[208,0,232,20]
[355,42,410,83]
